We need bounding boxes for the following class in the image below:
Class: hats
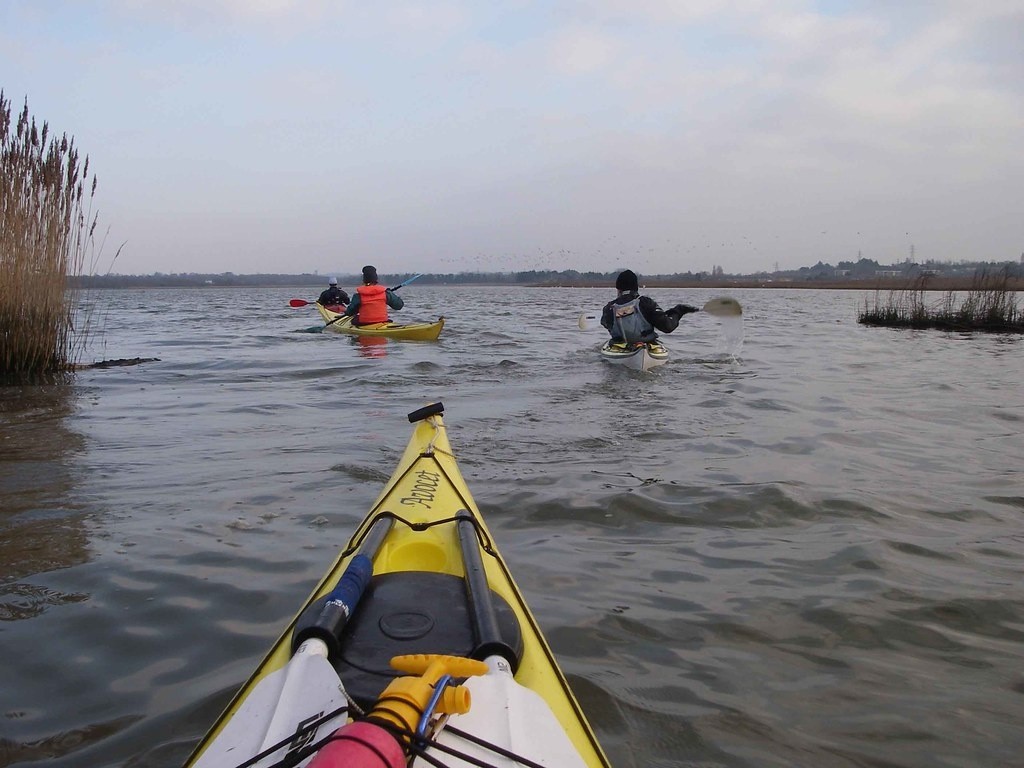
[616,270,638,291]
[329,279,337,284]
[362,266,378,280]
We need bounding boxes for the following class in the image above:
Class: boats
[315,300,445,341]
[600,339,670,372]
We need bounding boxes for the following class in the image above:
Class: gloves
[386,288,390,291]
[676,304,699,314]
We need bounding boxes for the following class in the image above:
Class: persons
[317,277,350,306]
[345,265,404,326]
[601,270,699,346]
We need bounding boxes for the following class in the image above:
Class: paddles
[411,509,588,767]
[306,273,424,332]
[288,299,351,307]
[578,297,742,330]
[191,513,396,768]
